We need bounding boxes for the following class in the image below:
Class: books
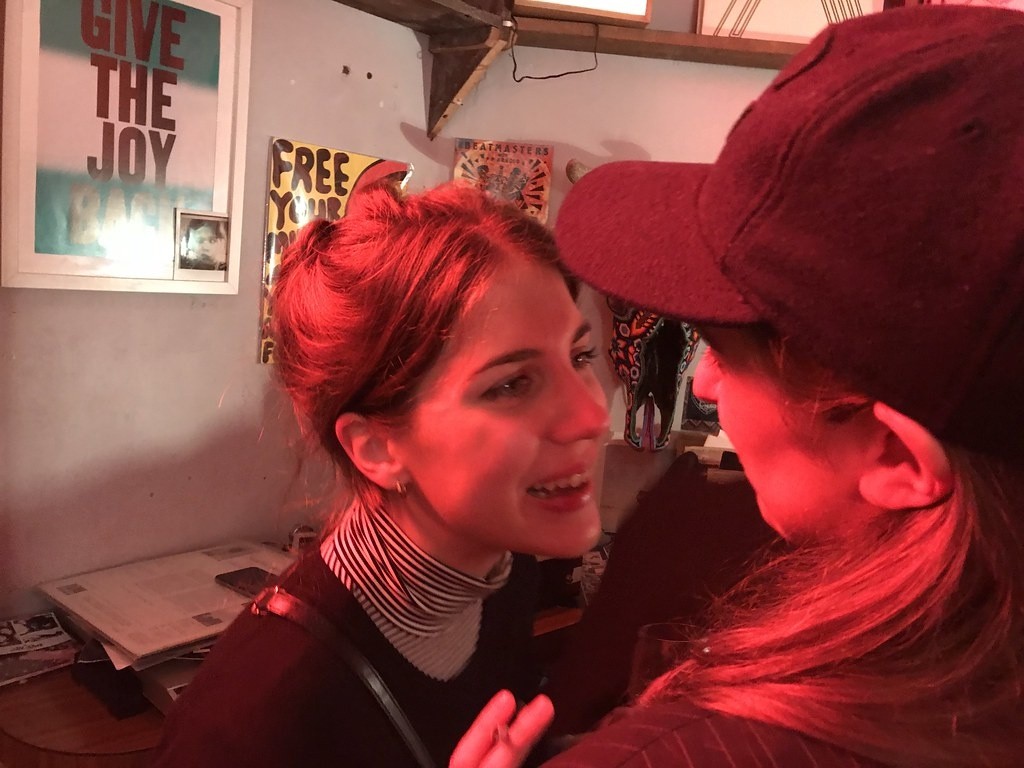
[0,637,84,686]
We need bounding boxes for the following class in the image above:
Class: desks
[0,671,162,768]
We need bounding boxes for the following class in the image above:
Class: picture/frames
[1,0,252,296]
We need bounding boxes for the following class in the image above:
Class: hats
[554,5,1024,462]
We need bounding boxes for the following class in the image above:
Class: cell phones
[214,567,280,599]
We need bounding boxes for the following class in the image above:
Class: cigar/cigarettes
[496,721,508,741]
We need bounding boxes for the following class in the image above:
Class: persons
[152,179,614,768]
[178,219,227,270]
[537,4,1024,768]
